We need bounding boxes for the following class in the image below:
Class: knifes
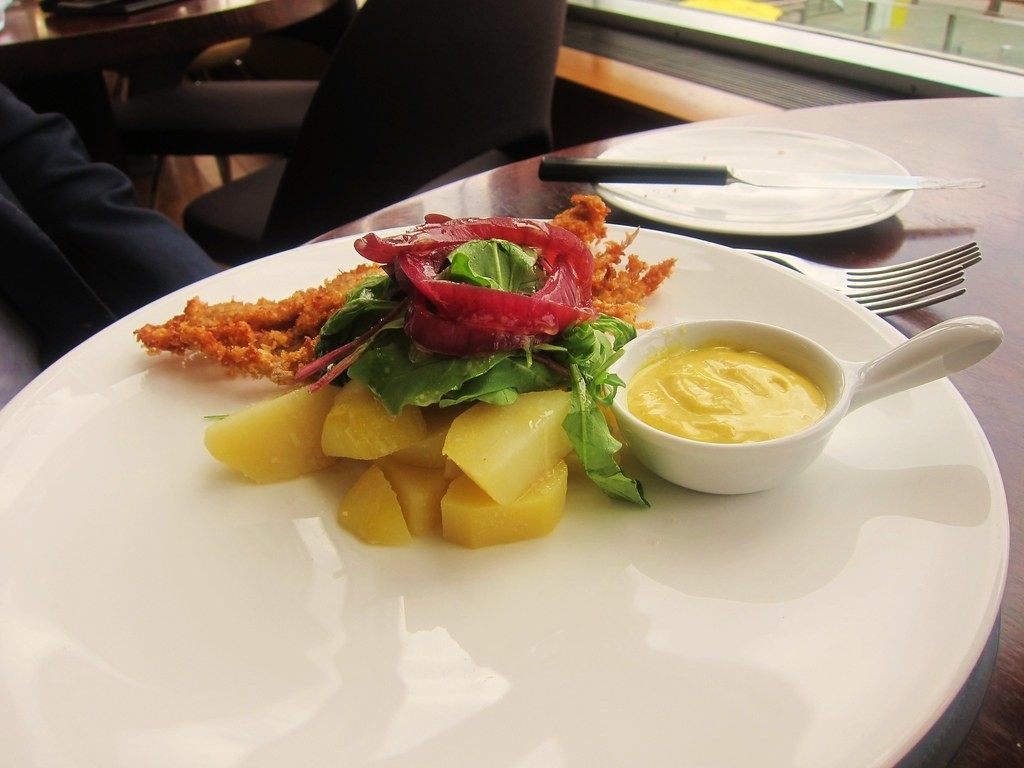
[536,154,990,193]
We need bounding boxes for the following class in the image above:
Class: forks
[737,240,983,317]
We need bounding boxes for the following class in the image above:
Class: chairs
[109,0,568,267]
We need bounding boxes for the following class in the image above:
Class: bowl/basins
[604,314,1009,495]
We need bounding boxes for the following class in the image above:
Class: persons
[0,0,565,375]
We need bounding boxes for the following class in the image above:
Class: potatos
[202,374,579,546]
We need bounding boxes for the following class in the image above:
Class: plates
[0,215,1011,767]
[595,124,914,237]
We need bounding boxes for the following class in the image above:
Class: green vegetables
[295,232,650,508]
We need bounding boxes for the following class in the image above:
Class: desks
[325,96,1024,768]
[0,0,339,87]
